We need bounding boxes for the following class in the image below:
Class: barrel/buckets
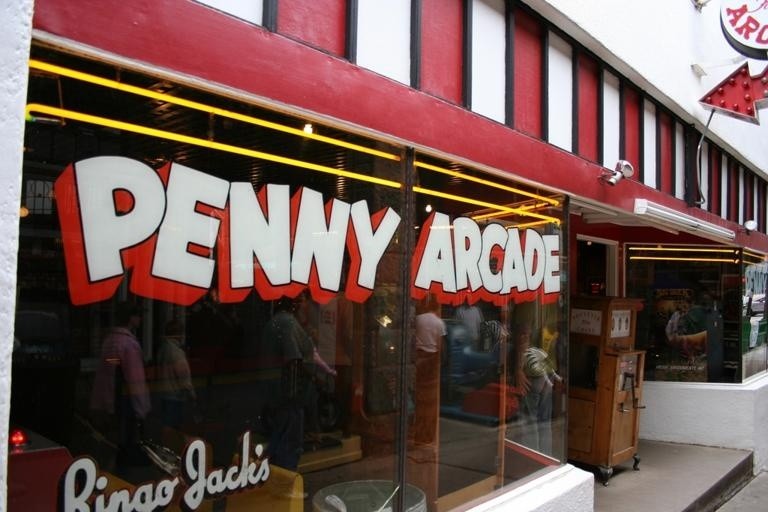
[740,317,767,354]
[313,479,427,512]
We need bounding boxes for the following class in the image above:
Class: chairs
[224,454,305,511]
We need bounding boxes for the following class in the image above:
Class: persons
[151,319,205,440]
[663,295,691,351]
[476,304,513,354]
[87,300,151,475]
[451,295,486,354]
[411,291,448,447]
[295,306,340,451]
[251,290,335,502]
[679,290,723,383]
[295,288,357,450]
[511,294,558,459]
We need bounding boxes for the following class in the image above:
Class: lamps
[740,218,758,237]
[597,156,637,190]
[633,198,738,246]
